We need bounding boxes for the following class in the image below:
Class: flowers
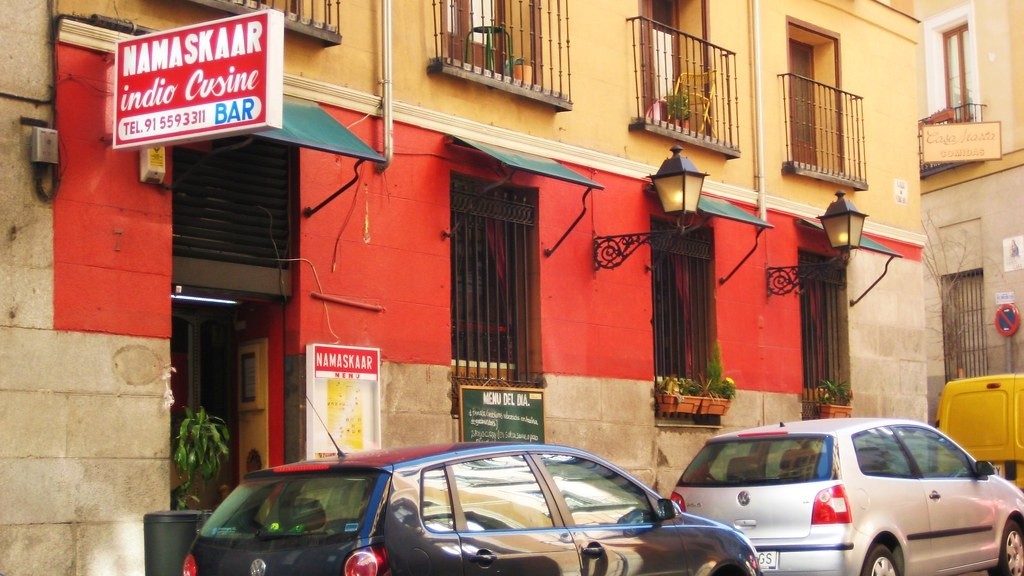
[817,380,852,406]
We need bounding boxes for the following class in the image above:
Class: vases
[816,403,854,419]
[931,109,955,123]
[918,119,933,130]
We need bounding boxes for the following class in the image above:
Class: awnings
[647,182,775,284]
[156,101,388,218]
[439,132,605,258]
[795,217,904,306]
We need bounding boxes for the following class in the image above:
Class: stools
[465,26,513,77]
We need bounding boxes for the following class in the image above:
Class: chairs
[780,450,817,478]
[278,495,325,533]
[668,71,718,138]
[726,457,767,481]
[858,447,891,476]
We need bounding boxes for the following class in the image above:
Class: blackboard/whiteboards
[459,385,545,444]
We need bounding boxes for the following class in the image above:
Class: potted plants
[656,340,736,415]
[665,89,691,128]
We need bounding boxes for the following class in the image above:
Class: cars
[670,417,1024,576]
[181,442,760,576]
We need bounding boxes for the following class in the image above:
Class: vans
[936,374,1024,491]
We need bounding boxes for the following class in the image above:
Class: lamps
[766,190,869,298]
[593,145,711,271]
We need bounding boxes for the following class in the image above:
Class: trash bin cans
[144,509,212,576]
